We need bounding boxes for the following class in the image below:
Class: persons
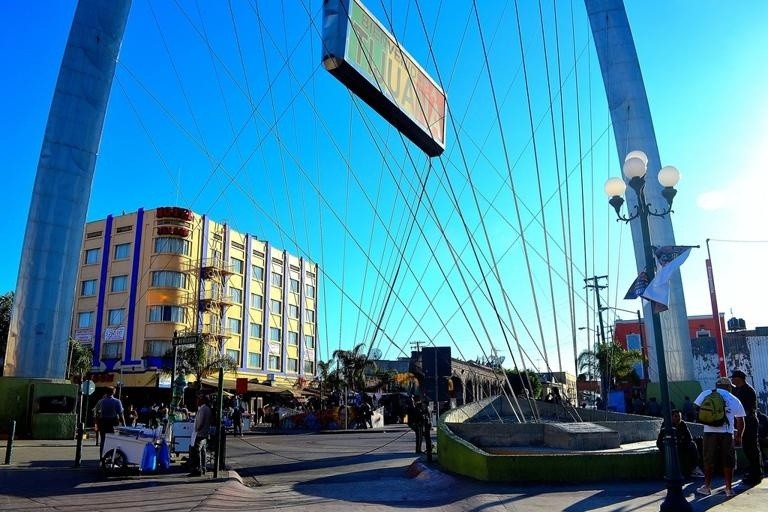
[544,387,561,403]
[93,385,244,477]
[355,400,374,430]
[631,370,764,497]
[408,400,433,455]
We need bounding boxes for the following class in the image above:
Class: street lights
[605,150,695,512]
[579,325,600,343]
[598,306,648,383]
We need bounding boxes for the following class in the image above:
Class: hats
[728,371,748,378]
[716,377,736,387]
[195,393,207,402]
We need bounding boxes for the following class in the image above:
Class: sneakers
[680,476,685,480]
[725,488,735,496]
[742,473,763,483]
[415,451,424,454]
[187,468,206,476]
[690,467,705,477]
[696,485,711,495]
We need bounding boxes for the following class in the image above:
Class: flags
[623,246,693,314]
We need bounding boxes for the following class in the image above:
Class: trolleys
[99,419,211,474]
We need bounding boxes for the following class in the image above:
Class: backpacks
[407,407,415,431]
[698,392,725,426]
